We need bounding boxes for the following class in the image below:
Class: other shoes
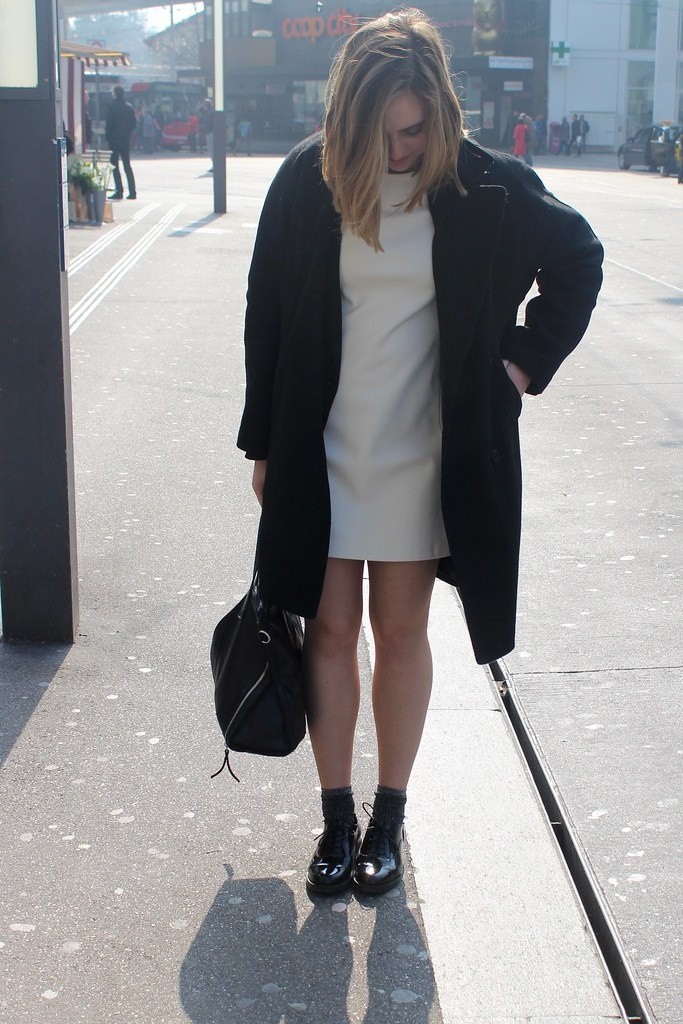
[125,191,137,201]
[107,189,123,200]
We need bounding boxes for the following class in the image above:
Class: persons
[237,13,605,901]
[202,99,216,175]
[556,113,590,157]
[674,130,683,183]
[106,86,136,199]
[133,107,252,157]
[514,112,547,167]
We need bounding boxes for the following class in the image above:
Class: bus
[83,81,210,151]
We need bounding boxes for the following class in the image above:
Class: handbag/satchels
[208,573,311,780]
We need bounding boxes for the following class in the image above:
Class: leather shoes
[305,815,362,896]
[349,816,404,897]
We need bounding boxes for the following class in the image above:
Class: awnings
[59,41,132,66]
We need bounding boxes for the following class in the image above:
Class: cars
[617,121,683,177]
